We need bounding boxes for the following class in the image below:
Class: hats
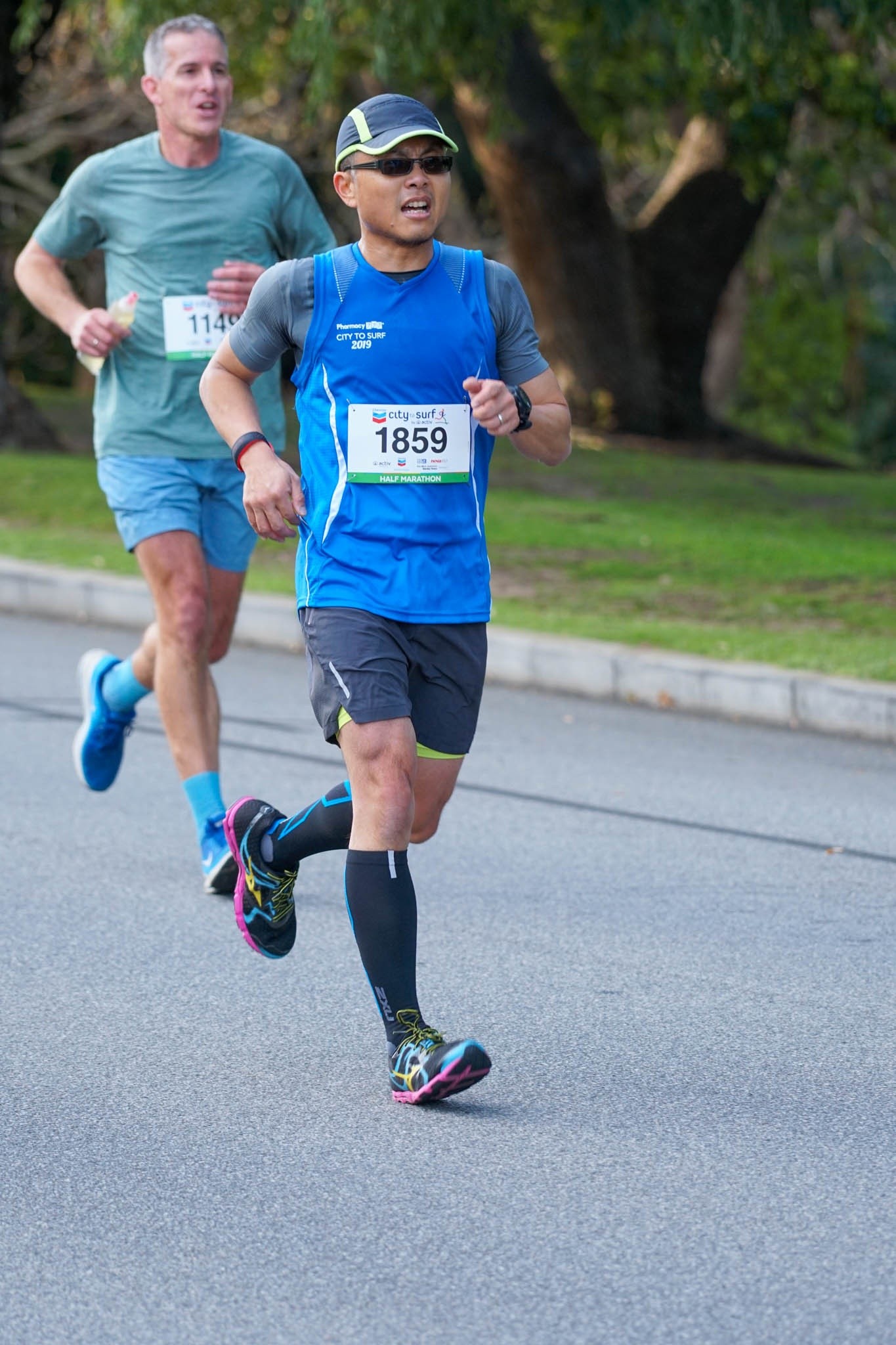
[333,93,460,170]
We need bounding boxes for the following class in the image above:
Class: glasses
[340,154,453,177]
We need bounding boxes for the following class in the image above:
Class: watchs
[507,384,533,432]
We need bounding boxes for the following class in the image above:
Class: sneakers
[387,1008,493,1107]
[199,819,240,891]
[73,649,137,792]
[225,797,298,958]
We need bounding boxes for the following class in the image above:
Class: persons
[199,92,573,1110]
[15,13,340,897]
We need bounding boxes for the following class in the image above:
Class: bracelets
[231,431,274,473]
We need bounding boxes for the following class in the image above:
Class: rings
[92,338,99,347]
[497,414,504,426]
